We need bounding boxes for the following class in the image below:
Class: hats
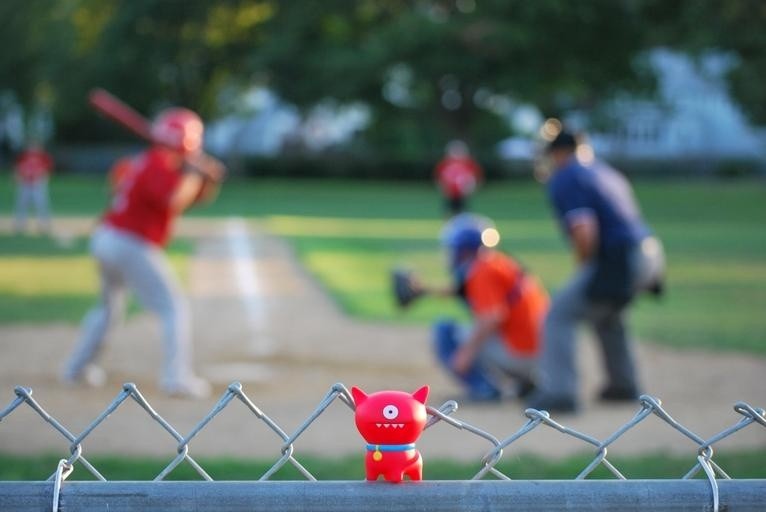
[542,130,576,155]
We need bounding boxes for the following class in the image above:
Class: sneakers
[462,384,638,412]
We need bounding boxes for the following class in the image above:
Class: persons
[392,212,552,401]
[527,129,664,413]
[12,138,54,235]
[52,105,224,399]
[436,140,481,214]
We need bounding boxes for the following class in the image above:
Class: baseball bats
[90,89,220,176]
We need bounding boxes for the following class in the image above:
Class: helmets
[150,107,204,156]
[438,212,483,290]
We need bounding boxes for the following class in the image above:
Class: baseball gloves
[393,269,421,307]
